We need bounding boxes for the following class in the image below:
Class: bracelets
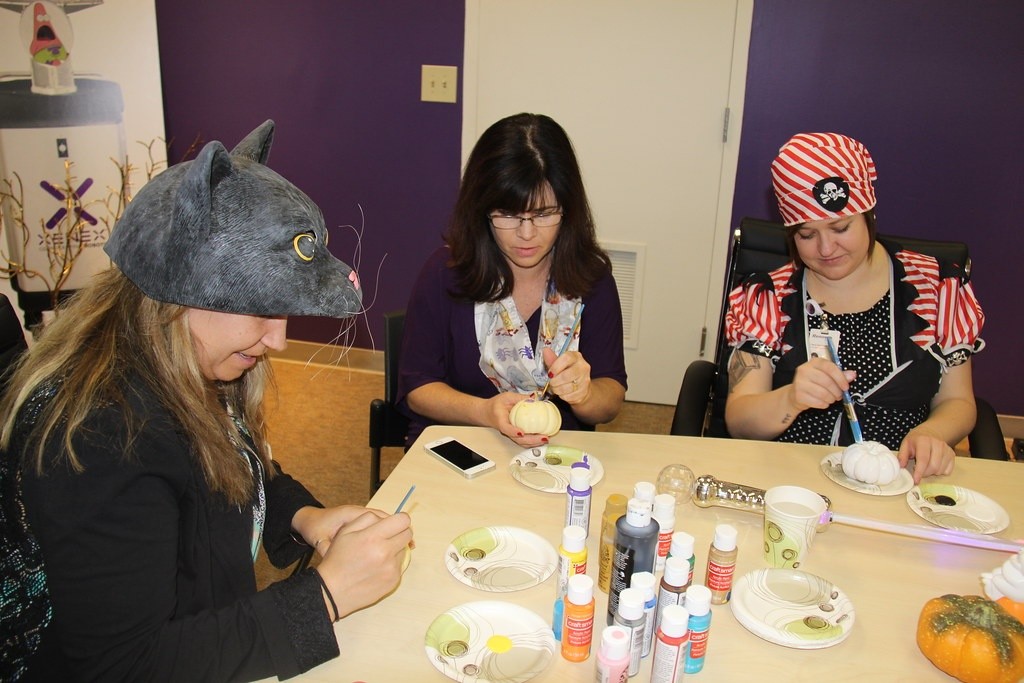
[313,568,339,623]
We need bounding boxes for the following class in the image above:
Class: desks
[274,425,1023,683]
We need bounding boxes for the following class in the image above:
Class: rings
[314,538,330,549]
[572,380,578,392]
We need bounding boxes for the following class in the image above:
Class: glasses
[487,212,562,229]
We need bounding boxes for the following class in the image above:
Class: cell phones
[424,437,497,479]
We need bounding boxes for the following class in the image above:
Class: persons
[392,112,629,450]
[722,131,986,486]
[2,118,412,682]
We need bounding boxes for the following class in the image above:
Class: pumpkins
[510,390,562,437]
[915,593,1024,683]
[403,544,411,573]
[841,440,900,486]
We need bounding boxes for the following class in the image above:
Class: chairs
[1,292,31,393]
[668,215,1010,460]
[370,307,411,498]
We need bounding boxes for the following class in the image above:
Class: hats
[102,119,364,319]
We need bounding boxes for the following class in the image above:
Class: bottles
[552,462,739,683]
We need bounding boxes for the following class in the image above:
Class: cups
[762,485,827,572]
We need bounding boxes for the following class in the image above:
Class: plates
[426,600,556,683]
[820,451,915,496]
[446,526,558,592]
[730,568,856,649]
[907,483,1010,534]
[509,445,604,494]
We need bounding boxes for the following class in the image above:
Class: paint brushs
[828,337,864,447]
[393,485,415,515]
[539,305,585,399]
